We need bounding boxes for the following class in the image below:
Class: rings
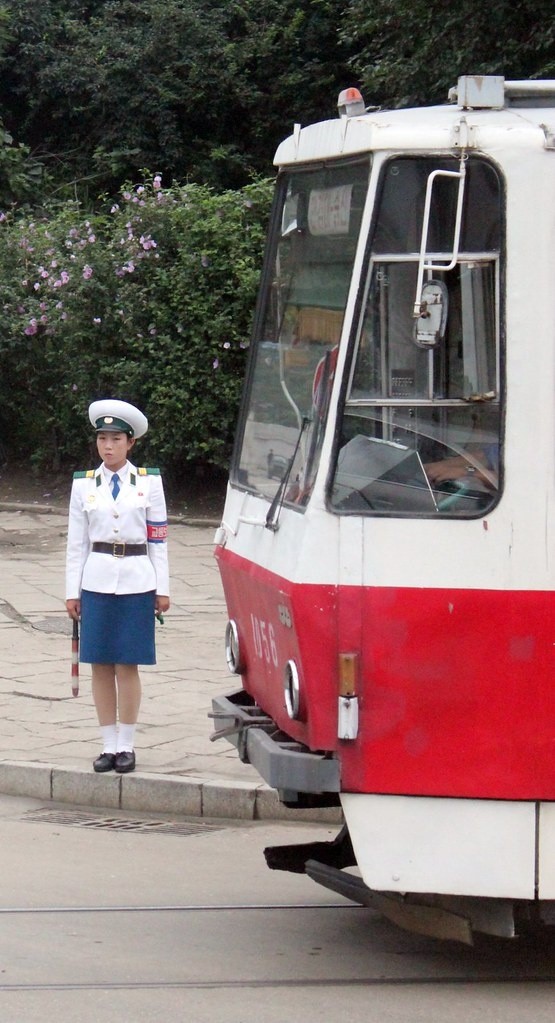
[158,607,163,612]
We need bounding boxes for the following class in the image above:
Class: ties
[111,474,120,501]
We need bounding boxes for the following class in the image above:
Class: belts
[92,542,147,558]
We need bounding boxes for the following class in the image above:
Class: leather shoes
[93,753,116,772]
[115,749,135,772]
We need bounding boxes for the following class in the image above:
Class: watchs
[464,466,476,476]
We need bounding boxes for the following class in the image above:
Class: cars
[268,454,288,481]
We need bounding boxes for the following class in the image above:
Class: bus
[209,76,555,943]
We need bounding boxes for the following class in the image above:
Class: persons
[419,410,500,489]
[65,400,170,773]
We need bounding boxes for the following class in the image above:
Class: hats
[89,399,148,439]
